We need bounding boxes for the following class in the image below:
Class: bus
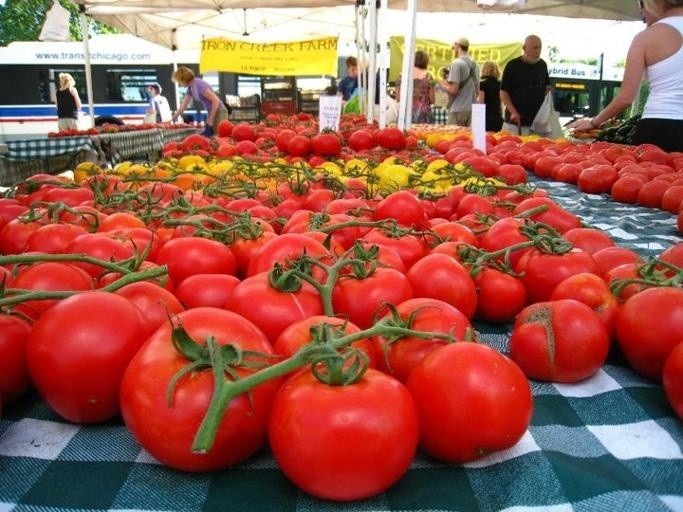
[546,64,637,133]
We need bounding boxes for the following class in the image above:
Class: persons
[169,66,229,137]
[53,72,82,132]
[335,55,369,107]
[436,36,483,129]
[477,61,504,133]
[146,82,173,127]
[497,33,554,144]
[394,49,438,129]
[566,0,683,154]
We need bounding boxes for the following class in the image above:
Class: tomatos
[419,133,682,229]
[0,162,682,503]
[45,119,205,138]
[387,118,571,145]
[157,109,446,165]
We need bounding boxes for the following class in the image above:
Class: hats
[452,38,468,49]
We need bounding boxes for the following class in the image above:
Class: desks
[0,124,206,182]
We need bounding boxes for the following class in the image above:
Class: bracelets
[590,115,600,130]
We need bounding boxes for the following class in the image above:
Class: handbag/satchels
[143,112,157,123]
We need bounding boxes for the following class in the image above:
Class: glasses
[638,0,643,9]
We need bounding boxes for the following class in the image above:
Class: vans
[0,40,178,148]
